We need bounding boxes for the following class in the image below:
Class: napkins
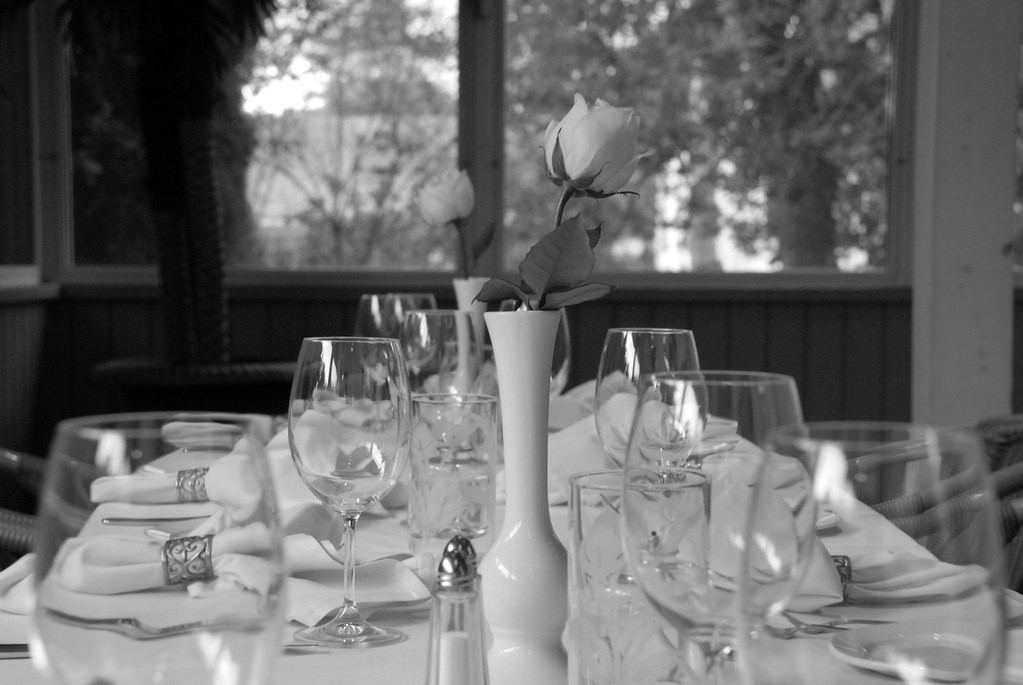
[61,410,433,626]
[497,371,740,507]
[790,541,992,611]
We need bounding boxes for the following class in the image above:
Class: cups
[567,465,719,685]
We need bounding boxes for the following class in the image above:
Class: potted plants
[53,1,297,415]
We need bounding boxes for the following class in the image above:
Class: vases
[477,311,568,635]
[453,278,489,373]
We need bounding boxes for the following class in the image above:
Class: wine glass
[284,334,411,649]
[498,298,572,402]
[620,370,817,685]
[594,328,707,469]
[29,410,284,684]
[396,308,482,442]
[730,419,1011,684]
[353,292,439,406]
[399,393,503,601]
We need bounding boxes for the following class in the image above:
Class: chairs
[846,415,1023,594]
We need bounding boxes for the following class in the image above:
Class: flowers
[415,170,499,279]
[470,91,659,311]
